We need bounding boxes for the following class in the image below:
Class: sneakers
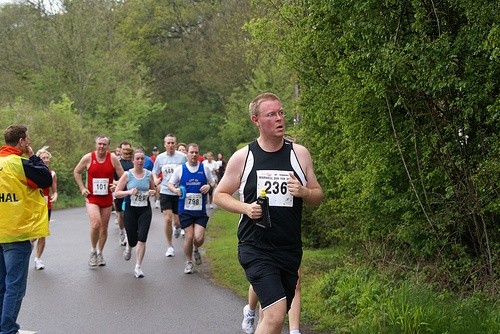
[134,267,145,278]
[193,251,202,265]
[96,253,106,266]
[35,257,45,270]
[89,250,98,266]
[165,246,175,257]
[123,243,132,261]
[174,224,180,238]
[119,230,127,246]
[241,304,256,334]
[180,229,186,237]
[184,261,196,274]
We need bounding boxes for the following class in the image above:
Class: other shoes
[16,329,38,334]
[155,202,159,208]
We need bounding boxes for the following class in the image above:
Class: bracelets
[209,184,212,188]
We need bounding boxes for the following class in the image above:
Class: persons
[74,135,124,267]
[0,124,58,334]
[152,135,187,257]
[114,149,157,279]
[213,93,322,334]
[177,142,186,154]
[144,155,154,172]
[198,152,226,209]
[112,146,121,224]
[168,143,216,274]
[149,146,161,209]
